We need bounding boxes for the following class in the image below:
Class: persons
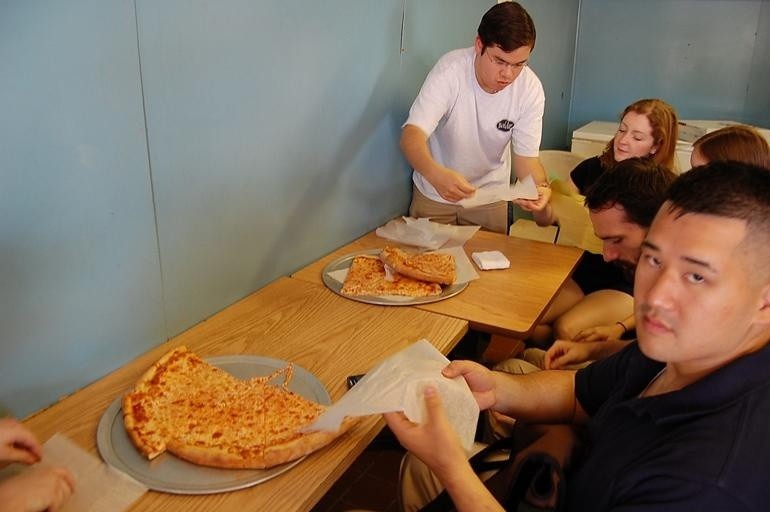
[0,415,76,511]
[486,98,768,441]
[382,159,769,511]
[398,1,551,362]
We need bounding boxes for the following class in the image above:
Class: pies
[340,255,443,297]
[121,344,364,472]
[379,243,457,289]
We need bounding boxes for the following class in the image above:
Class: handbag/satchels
[498,416,576,511]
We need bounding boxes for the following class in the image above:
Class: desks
[1,120,770,512]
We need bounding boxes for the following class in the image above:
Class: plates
[97,354,332,497]
[323,249,471,306]
